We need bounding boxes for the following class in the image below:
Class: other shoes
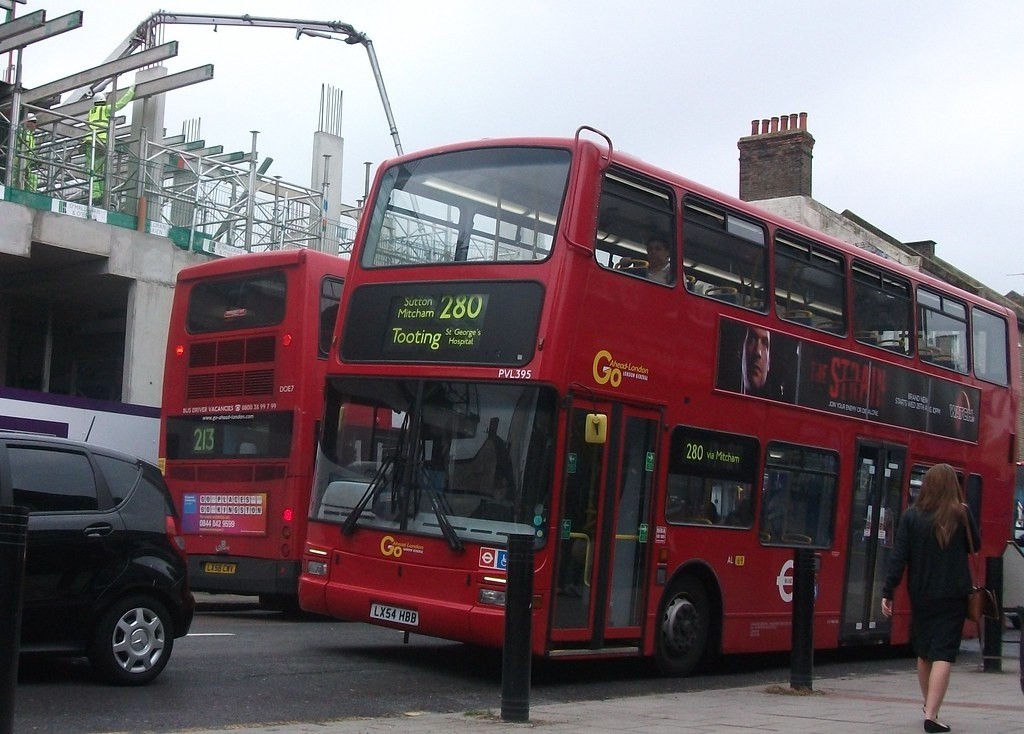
[924,719,951,733]
[923,707,938,719]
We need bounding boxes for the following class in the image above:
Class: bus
[295,134,1024,684]
[151,245,408,619]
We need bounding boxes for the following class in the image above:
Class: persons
[882,464,982,733]
[737,325,786,400]
[724,499,777,545]
[13,113,38,192]
[620,236,669,283]
[85,83,138,205]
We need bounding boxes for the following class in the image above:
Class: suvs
[1,426,198,687]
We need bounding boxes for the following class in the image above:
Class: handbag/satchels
[966,587,999,622]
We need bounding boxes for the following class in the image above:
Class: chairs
[685,516,812,545]
[602,259,981,379]
